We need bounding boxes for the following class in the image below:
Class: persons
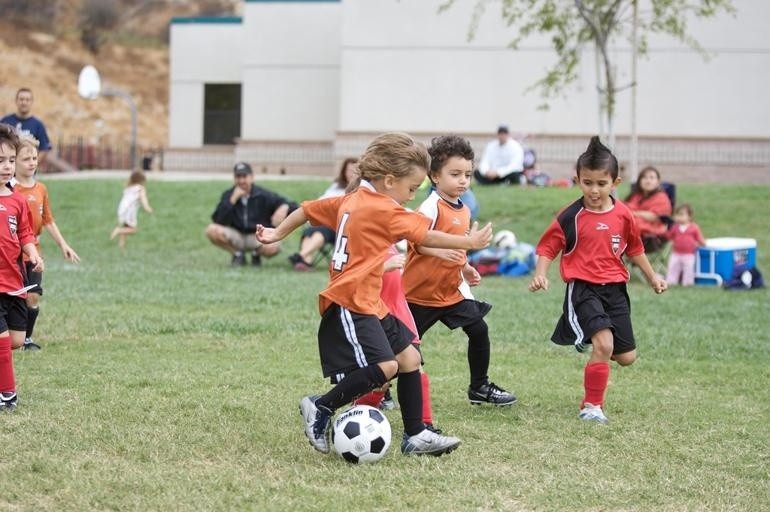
[474,126,527,187]
[353,203,442,439]
[255,133,491,456]
[624,167,671,240]
[10,136,80,349]
[662,205,704,286]
[0,120,45,414]
[110,170,154,248]
[1,88,51,165]
[204,161,297,266]
[381,136,518,408]
[526,136,667,427]
[288,157,360,270]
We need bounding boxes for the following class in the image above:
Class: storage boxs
[694,235,757,285]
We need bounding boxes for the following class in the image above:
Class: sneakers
[579,403,608,424]
[0,392,16,411]
[575,343,591,352]
[379,390,395,409]
[468,383,517,406]
[232,253,313,269]
[401,423,461,456]
[299,395,335,453]
[24,338,40,352]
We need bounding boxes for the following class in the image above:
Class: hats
[234,164,250,176]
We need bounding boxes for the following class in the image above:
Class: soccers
[331,406,391,463]
